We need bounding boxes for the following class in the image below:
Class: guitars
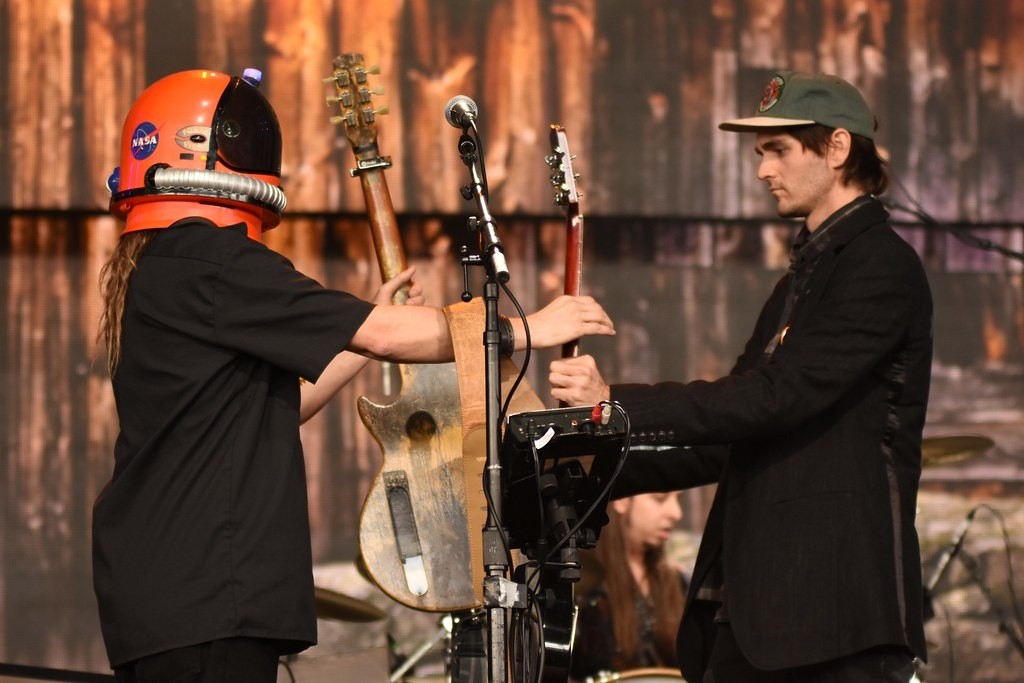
[320,51,547,613]
[546,123,583,653]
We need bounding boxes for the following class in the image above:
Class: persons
[546,70,934,683]
[90,69,616,683]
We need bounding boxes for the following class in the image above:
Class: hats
[719,70,876,138]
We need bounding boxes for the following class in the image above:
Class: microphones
[927,509,977,591]
[445,95,479,129]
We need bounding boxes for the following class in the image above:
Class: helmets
[100,68,281,245]
